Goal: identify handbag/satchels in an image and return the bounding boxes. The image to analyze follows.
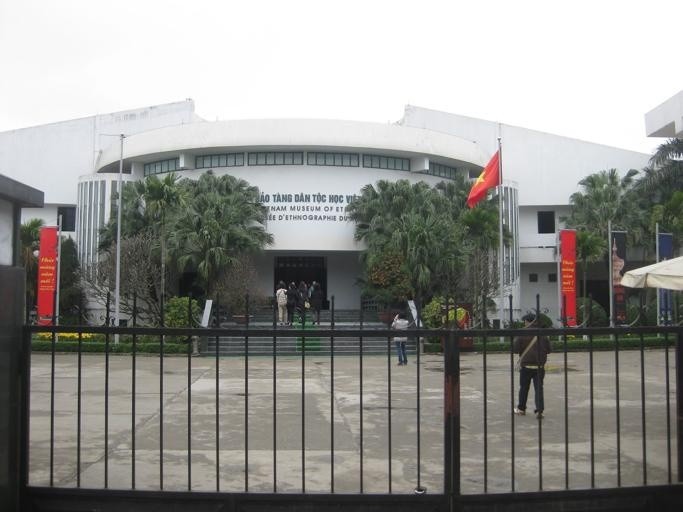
[305,301,311,309]
[514,359,522,372]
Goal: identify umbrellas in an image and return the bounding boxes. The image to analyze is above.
[620,254,682,293]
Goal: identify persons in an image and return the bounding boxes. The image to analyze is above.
[511,314,551,419]
[391,311,408,366]
[276,280,322,328]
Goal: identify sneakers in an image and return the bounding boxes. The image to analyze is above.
[398,361,407,366]
[514,407,545,419]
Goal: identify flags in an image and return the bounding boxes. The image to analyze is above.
[465,148,502,209]
